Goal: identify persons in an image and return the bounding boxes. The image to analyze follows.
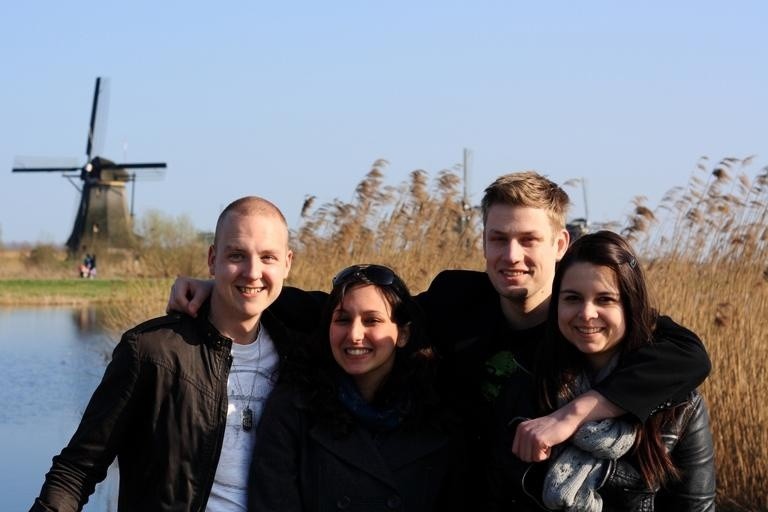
[163,167,714,512]
[78,252,98,279]
[486,226,718,512]
[248,263,494,509]
[26,195,321,512]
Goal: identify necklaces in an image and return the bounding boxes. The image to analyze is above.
[218,324,262,434]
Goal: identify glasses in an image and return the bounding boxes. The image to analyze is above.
[332,263,394,286]
